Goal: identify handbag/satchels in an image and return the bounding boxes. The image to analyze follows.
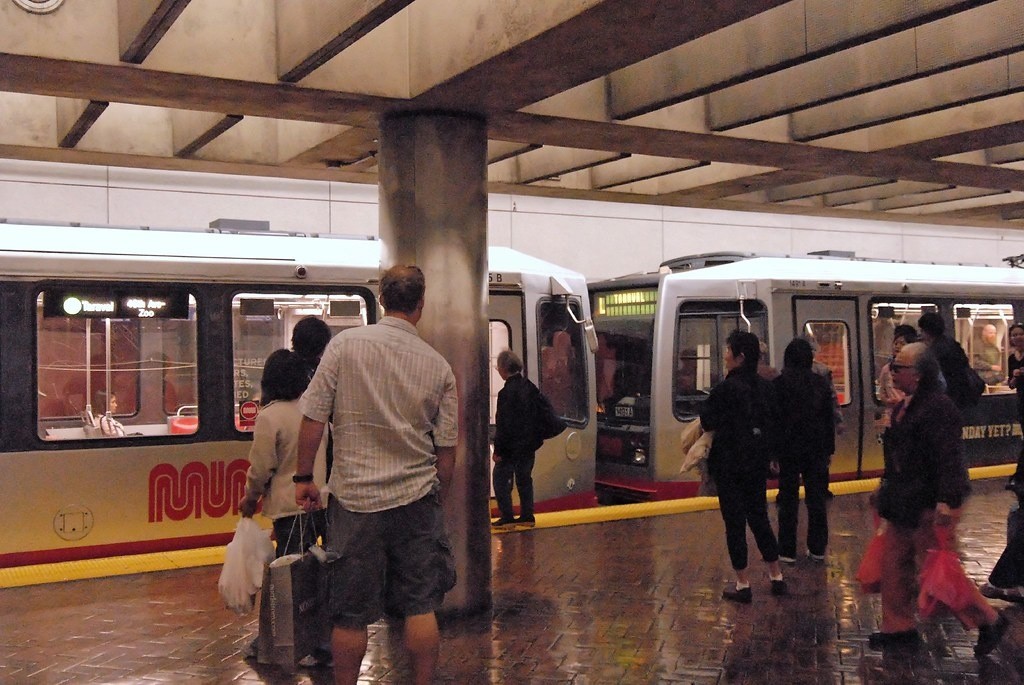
[257,506,328,666]
[917,523,999,630]
[217,516,277,614]
[856,511,890,593]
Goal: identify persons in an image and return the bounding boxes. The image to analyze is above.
[235,349,330,664]
[94,389,142,435]
[774,338,836,563]
[869,342,1009,658]
[292,317,334,483]
[699,331,786,602]
[981,504,1024,602]
[679,350,708,394]
[868,324,917,507]
[1005,325,1024,488]
[969,324,1003,384]
[295,266,458,685]
[492,350,545,528]
[920,312,973,496]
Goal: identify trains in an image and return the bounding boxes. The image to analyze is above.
[0,224,1024,569]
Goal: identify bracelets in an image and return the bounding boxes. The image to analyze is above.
[293,475,313,482]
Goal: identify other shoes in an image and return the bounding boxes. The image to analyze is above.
[806,549,824,564]
[297,654,321,666]
[722,582,753,604]
[981,580,1024,603]
[770,576,787,594]
[974,612,1009,655]
[868,629,918,647]
[516,516,535,526]
[1005,477,1022,489]
[490,517,516,528]
[243,637,258,655]
[777,553,797,565]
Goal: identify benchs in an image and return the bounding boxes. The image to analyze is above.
[78,400,259,444]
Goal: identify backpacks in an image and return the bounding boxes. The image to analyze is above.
[518,379,553,440]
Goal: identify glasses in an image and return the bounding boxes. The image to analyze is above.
[889,364,917,372]
[1008,322,1024,330]
